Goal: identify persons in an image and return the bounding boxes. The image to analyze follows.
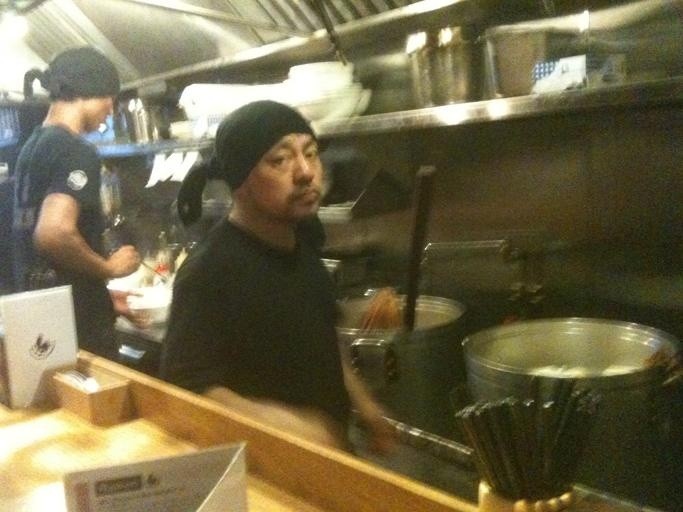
[13,45,143,360]
[158,101,390,452]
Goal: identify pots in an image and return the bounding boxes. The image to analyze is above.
[457,315,683,503]
[337,293,467,420]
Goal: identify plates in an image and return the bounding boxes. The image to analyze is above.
[317,206,354,225]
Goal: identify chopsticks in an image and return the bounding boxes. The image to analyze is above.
[455,374,597,501]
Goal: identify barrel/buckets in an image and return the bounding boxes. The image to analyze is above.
[404,37,492,108]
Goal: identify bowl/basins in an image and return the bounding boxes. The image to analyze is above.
[407,26,584,108]
[129,299,171,326]
[298,80,374,122]
